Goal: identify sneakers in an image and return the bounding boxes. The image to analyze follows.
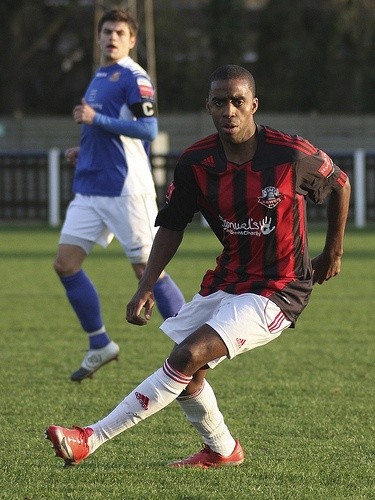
[70,341,120,382]
[166,438,245,469]
[45,423,93,466]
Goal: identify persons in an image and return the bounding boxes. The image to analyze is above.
[45,64,351,469]
[54,9,187,383]
